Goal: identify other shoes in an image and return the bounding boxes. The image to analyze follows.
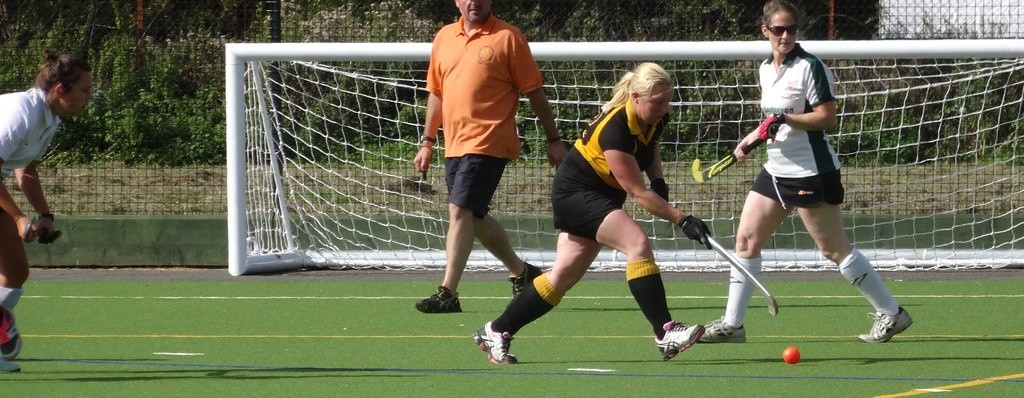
[0,360,21,371]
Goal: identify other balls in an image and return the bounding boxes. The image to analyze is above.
[782,346,801,364]
[705,231,780,318]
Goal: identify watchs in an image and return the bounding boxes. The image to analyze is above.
[420,135,436,143]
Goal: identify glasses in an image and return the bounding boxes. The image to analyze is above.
[766,24,799,36]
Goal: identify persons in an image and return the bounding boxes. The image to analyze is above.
[698,0,913,343]
[0,50,92,373]
[414,0,568,314]
[472,61,706,365]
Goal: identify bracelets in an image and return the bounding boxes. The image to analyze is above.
[421,146,433,152]
[549,137,561,142]
[15,215,26,222]
[38,213,54,221]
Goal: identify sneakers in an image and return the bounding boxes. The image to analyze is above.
[698,315,747,342]
[505,262,543,308]
[415,285,462,314]
[858,305,913,342]
[0,307,22,360]
[654,320,705,362]
[472,321,517,364]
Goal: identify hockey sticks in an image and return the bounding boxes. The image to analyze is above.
[691,124,780,184]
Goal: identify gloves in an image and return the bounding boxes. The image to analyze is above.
[651,178,668,202]
[677,215,713,251]
[758,113,784,141]
